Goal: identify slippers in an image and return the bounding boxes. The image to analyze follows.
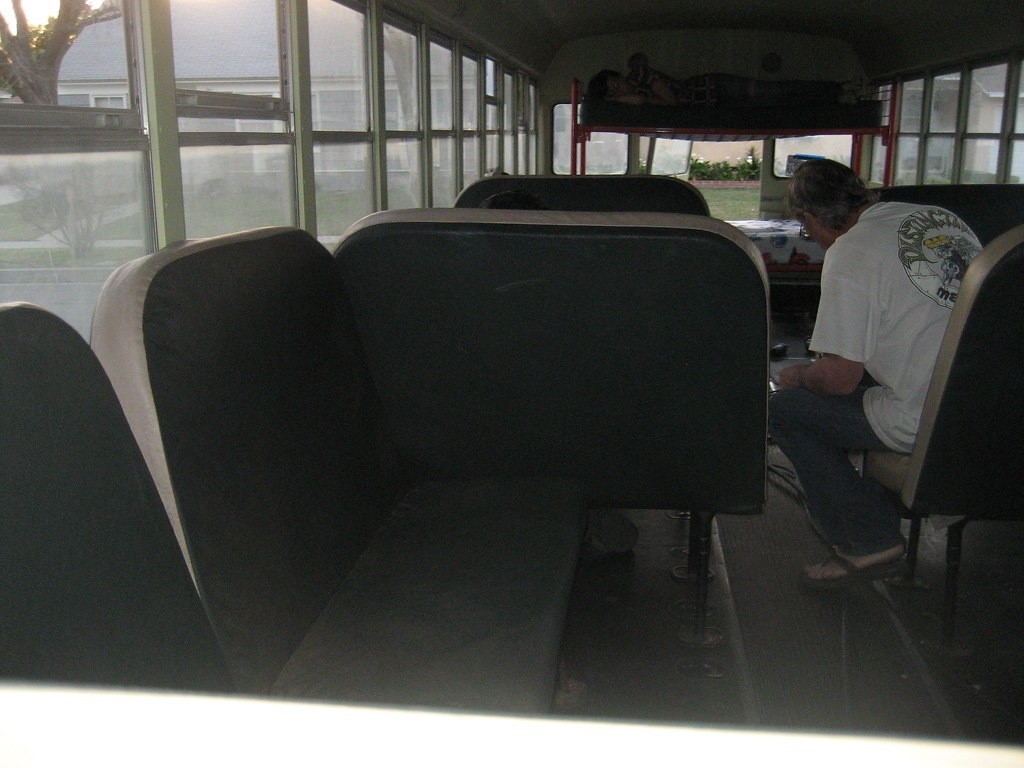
[806,550,909,589]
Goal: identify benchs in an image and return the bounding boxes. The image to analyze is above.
[0,176,773,717]
[862,184,1024,650]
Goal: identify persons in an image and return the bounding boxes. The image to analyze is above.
[766,159,986,567]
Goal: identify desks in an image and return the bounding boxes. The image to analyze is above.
[724,220,828,325]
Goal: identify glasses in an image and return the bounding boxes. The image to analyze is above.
[798,217,813,241]
